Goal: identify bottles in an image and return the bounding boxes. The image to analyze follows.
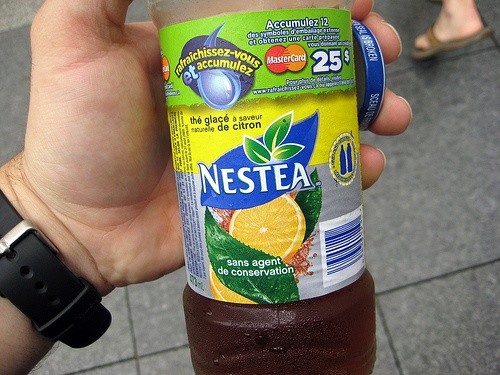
[146,0,378,375]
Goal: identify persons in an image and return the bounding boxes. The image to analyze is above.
[0,0,411,375]
[409,0,492,62]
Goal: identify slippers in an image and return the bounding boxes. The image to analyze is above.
[407,22,493,59]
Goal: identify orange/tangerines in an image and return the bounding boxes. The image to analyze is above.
[210,193,308,305]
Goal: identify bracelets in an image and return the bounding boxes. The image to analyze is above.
[0,190,114,347]
